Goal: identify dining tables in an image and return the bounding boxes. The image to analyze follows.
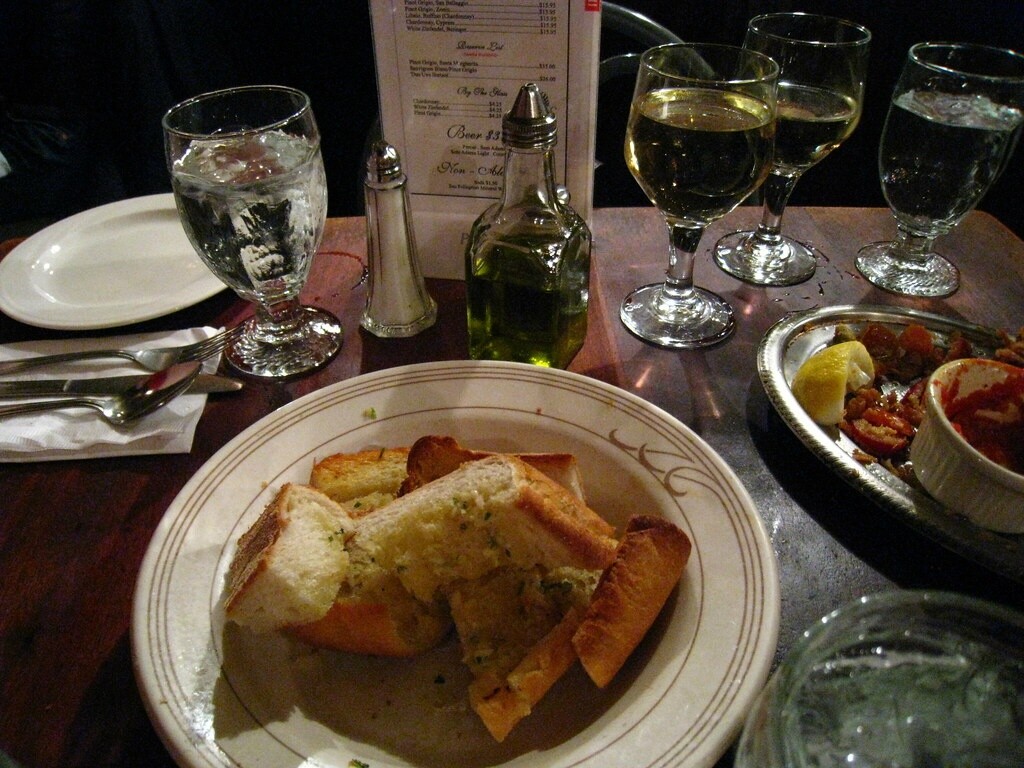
[0,205,1024,768]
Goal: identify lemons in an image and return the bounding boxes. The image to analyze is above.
[792,341,875,424]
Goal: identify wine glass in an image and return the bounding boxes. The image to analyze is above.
[855,44,1024,297]
[711,13,873,289]
[161,85,344,381]
[618,44,780,349]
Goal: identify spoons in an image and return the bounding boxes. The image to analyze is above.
[0,363,204,425]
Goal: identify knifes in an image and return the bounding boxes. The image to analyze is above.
[0,374,243,399]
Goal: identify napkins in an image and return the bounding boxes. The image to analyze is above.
[0,326,227,466]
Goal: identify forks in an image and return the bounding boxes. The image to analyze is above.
[0,319,248,375]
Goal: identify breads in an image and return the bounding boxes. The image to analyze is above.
[222,433,693,744]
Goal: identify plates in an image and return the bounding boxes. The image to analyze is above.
[757,305,1024,582]
[131,360,781,768]
[0,192,229,330]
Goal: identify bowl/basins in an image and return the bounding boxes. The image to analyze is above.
[910,358,1024,534]
[733,586,1024,768]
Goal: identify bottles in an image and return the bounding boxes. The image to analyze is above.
[358,141,438,338]
[463,84,592,370]
